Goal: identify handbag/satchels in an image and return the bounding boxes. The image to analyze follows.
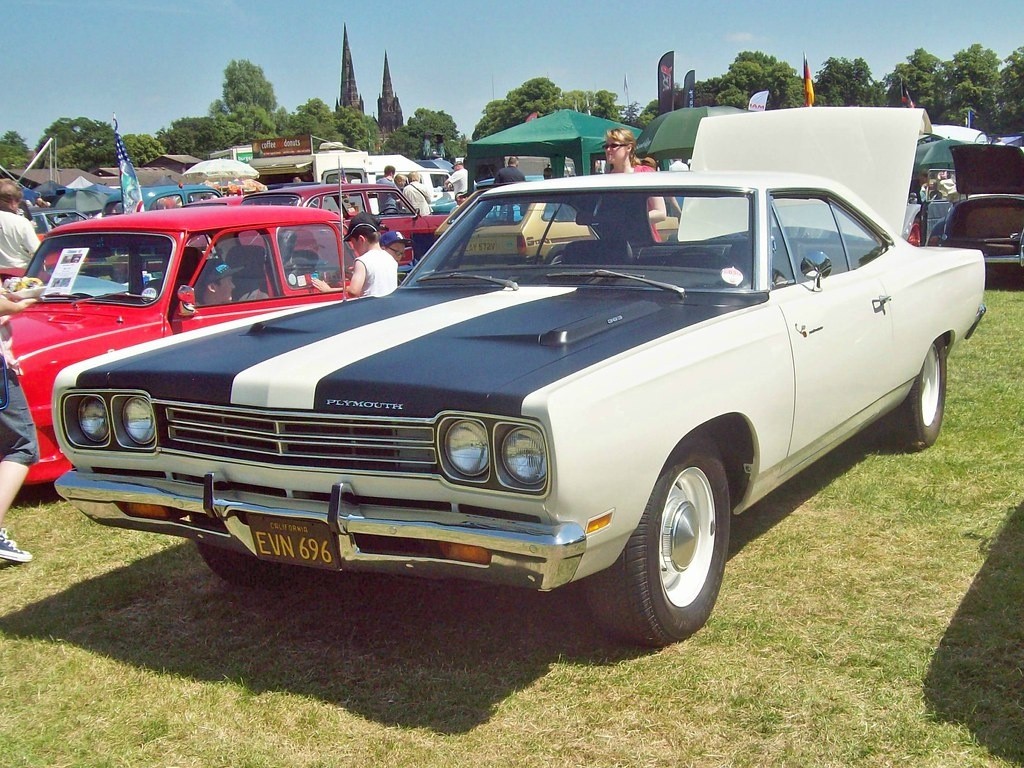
[0,355,10,411]
[424,194,431,205]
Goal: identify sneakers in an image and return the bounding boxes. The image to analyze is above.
[0,528,33,563]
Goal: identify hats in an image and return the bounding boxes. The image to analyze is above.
[380,230,414,250]
[344,211,380,242]
[642,157,657,169]
[196,259,244,287]
[452,161,463,169]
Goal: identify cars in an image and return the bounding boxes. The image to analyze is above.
[2,203,358,489]
[1,172,573,262]
[923,143,1024,266]
[51,106,987,646]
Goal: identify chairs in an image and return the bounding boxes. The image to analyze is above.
[224,244,266,300]
[562,239,635,266]
[160,243,205,312]
[634,242,735,271]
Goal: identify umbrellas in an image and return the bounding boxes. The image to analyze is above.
[182,158,267,197]
[913,138,975,171]
[633,106,752,161]
[30,175,117,213]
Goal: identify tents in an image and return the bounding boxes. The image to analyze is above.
[466,108,644,196]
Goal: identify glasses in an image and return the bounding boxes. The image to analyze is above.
[602,143,629,153]
[384,245,406,258]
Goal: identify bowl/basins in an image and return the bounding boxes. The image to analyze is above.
[3,286,48,301]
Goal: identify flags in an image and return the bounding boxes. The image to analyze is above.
[749,91,769,111]
[804,60,814,107]
[658,51,674,114]
[683,70,695,108]
[114,131,145,214]
[965,109,975,127]
[900,81,914,107]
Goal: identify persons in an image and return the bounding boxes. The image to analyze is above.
[450,192,468,214]
[0,179,43,562]
[293,172,313,182]
[493,156,526,183]
[445,161,476,195]
[576,128,690,243]
[374,165,443,215]
[543,168,552,179]
[165,246,242,304]
[379,231,415,264]
[909,170,951,205]
[343,212,398,297]
